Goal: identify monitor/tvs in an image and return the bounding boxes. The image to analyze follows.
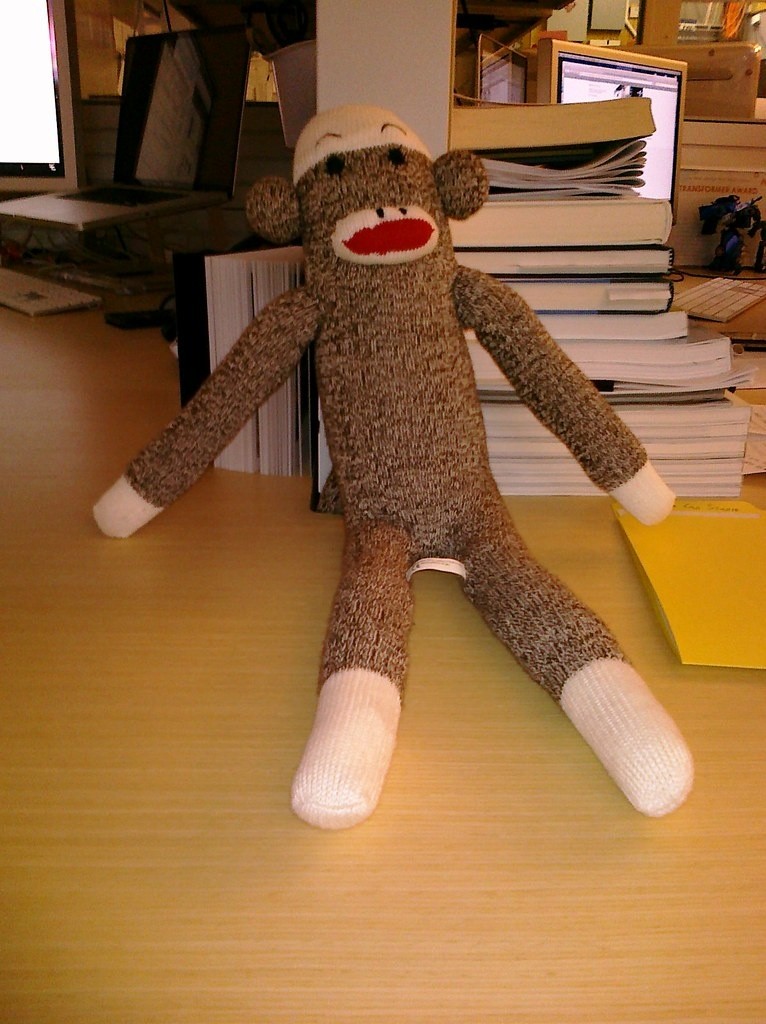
[549,39,688,227]
[473,33,529,108]
[0,0,86,191]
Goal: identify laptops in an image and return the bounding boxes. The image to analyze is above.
[0,23,258,233]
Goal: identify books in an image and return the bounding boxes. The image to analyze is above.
[449,97,758,498]
[171,246,313,479]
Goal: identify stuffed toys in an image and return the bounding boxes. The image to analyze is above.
[93,103,696,831]
